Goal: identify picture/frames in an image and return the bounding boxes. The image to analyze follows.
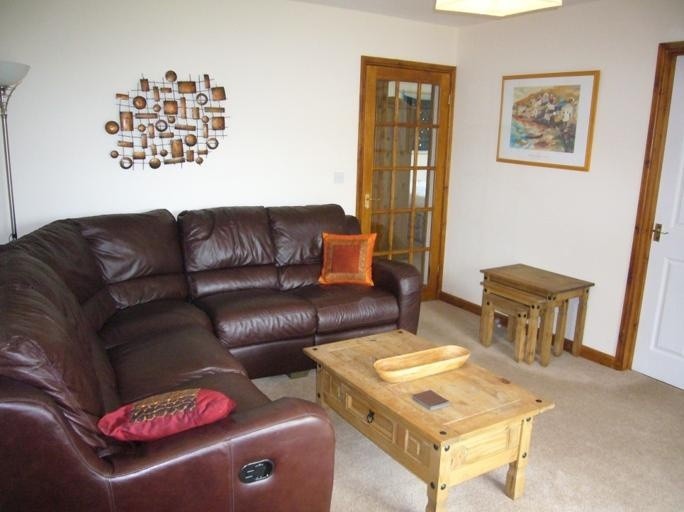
[495,70,599,172]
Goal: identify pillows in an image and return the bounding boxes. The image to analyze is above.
[318,227,376,289]
[97,388,235,440]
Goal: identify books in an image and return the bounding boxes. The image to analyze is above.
[412,389,451,413]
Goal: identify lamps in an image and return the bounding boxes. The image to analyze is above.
[0,61,30,241]
[430,1,563,19]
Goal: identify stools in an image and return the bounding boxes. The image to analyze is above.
[478,264,595,368]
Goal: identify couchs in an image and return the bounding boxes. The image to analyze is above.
[0,210,334,512]
[175,204,425,381]
